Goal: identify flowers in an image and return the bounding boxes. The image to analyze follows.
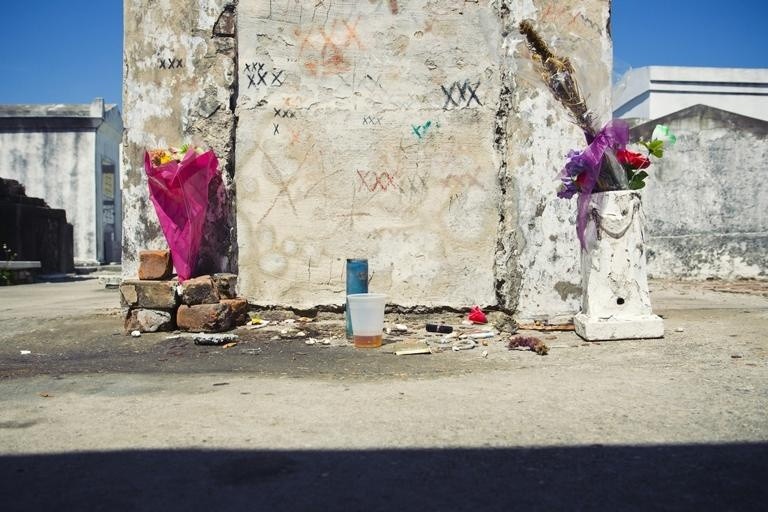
[144,144,219,282]
[557,119,676,199]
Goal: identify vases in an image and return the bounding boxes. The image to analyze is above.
[573,190,664,342]
